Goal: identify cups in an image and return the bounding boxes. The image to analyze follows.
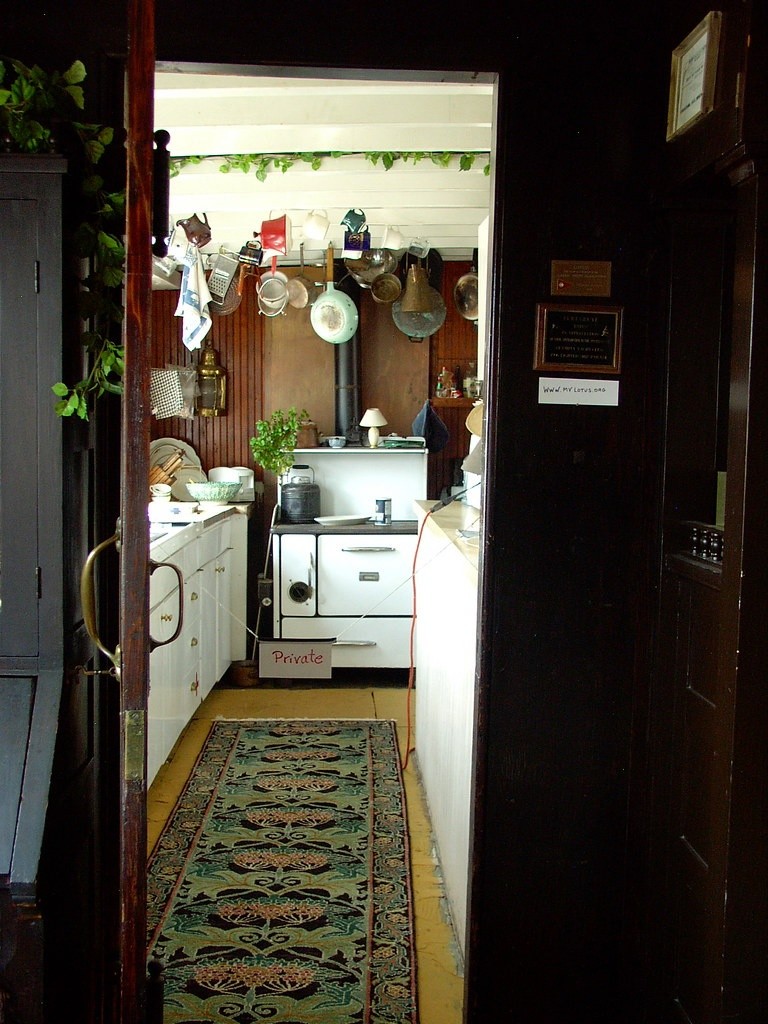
[343,225,372,252]
[153,254,177,277]
[149,483,172,495]
[239,240,264,267]
[176,210,212,248]
[339,208,367,235]
[406,236,432,258]
[164,223,176,248]
[380,225,405,251]
[374,497,393,526]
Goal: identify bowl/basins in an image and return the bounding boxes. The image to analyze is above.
[185,481,244,507]
[151,494,171,502]
[328,437,346,449]
[255,270,289,309]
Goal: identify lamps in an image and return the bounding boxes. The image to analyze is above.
[359,408,388,448]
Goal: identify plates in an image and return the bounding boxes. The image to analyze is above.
[315,515,373,526]
[149,436,207,502]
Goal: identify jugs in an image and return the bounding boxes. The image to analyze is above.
[299,207,330,241]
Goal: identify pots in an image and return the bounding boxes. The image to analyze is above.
[287,242,316,310]
[370,249,403,305]
[310,239,359,345]
[343,247,398,287]
[453,248,478,321]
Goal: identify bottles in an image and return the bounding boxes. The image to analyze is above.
[434,362,484,399]
[278,464,322,524]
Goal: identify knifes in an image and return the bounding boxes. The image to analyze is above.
[161,448,185,479]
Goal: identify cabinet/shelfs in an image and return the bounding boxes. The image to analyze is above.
[272,533,417,668]
[149,517,235,788]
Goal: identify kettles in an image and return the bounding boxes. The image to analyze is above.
[253,210,293,264]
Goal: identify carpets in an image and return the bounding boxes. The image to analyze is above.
[145,717,418,1024]
